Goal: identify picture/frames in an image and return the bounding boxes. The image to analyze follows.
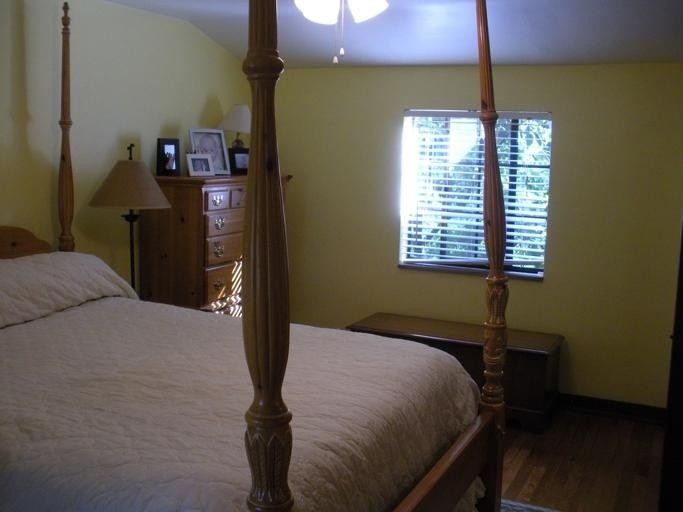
[226,147,251,177]
[154,137,181,179]
[189,129,232,177]
[185,152,216,179]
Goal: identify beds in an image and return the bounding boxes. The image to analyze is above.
[0,1,517,511]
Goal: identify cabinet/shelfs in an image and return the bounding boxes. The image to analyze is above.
[133,174,293,318]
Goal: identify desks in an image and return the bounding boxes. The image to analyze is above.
[344,309,568,424]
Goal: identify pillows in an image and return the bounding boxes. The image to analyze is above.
[0,252,121,329]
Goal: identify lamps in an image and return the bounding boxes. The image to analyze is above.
[215,102,254,146]
[290,0,391,72]
[87,143,175,299]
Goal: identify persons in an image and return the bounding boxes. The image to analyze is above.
[193,159,210,171]
[165,150,175,169]
[198,135,221,170]
